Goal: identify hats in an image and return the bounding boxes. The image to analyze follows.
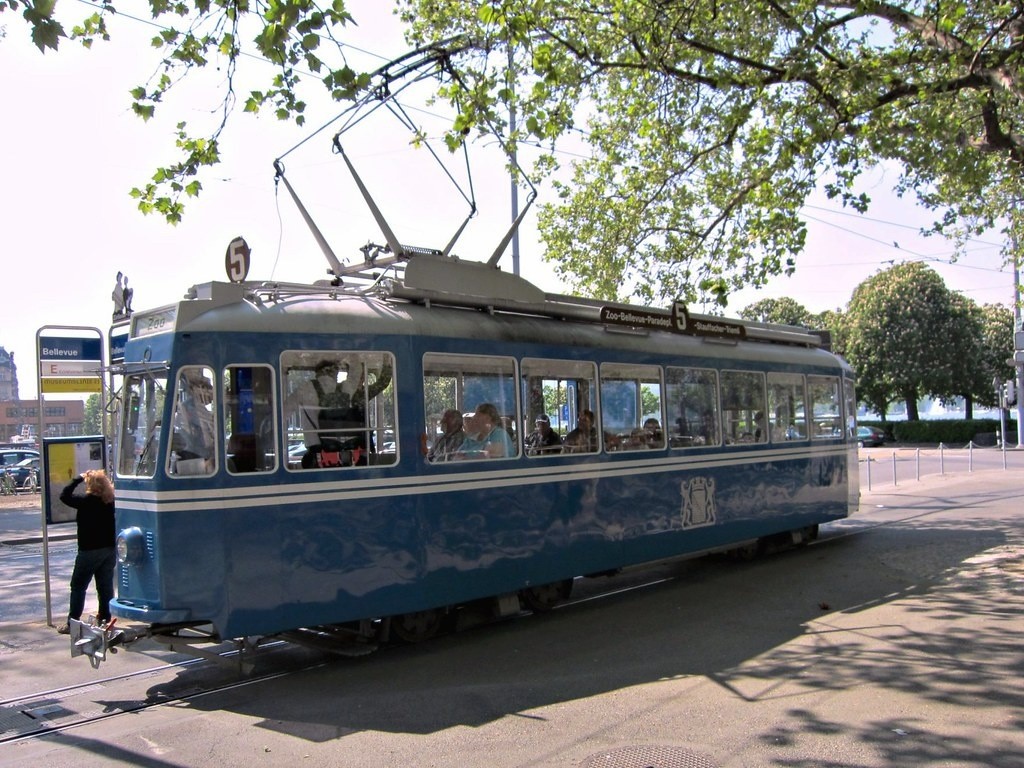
[535,414,549,422]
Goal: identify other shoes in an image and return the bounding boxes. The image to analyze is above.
[57,624,71,634]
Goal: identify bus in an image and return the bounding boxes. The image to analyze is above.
[69,237,861,680]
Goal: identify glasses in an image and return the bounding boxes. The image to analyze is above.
[577,418,589,424]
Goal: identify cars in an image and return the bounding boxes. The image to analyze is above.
[0,457,40,491]
[857,425,886,448]
[0,450,40,469]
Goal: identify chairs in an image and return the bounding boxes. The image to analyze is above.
[227,433,266,472]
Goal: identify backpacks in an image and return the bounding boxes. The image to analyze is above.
[303,380,359,453]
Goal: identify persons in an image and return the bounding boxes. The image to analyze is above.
[787,423,794,440]
[428,402,517,462]
[524,414,563,456]
[669,410,715,447]
[57,469,116,633]
[604,418,665,451]
[174,377,216,475]
[564,409,598,453]
[285,351,393,468]
[725,411,766,444]
